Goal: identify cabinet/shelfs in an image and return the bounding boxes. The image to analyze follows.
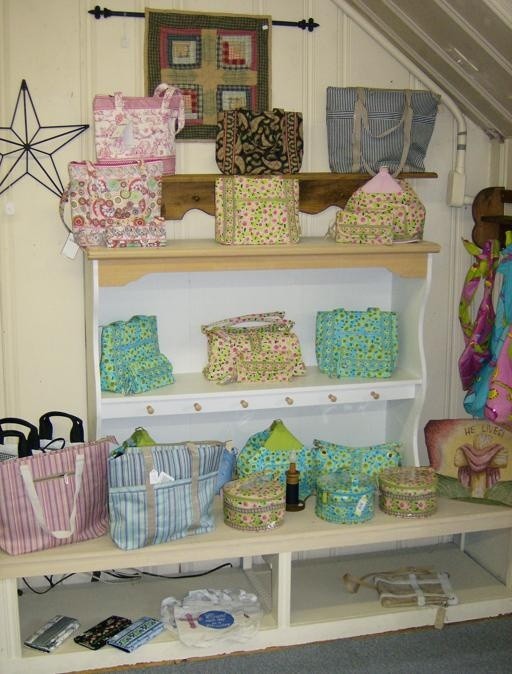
[82,238,441,469]
[0,491,512,674]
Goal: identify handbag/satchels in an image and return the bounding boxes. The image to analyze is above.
[213,103,305,246]
[315,466,378,523]
[378,467,442,517]
[343,565,459,629]
[214,438,236,496]
[462,250,511,428]
[236,419,316,504]
[107,426,223,550]
[219,469,287,533]
[200,310,308,384]
[66,94,185,250]
[312,436,404,506]
[326,85,442,246]
[99,314,173,394]
[1,411,119,555]
[316,306,400,379]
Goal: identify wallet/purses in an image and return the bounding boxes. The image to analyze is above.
[73,615,166,654]
[24,614,81,653]
